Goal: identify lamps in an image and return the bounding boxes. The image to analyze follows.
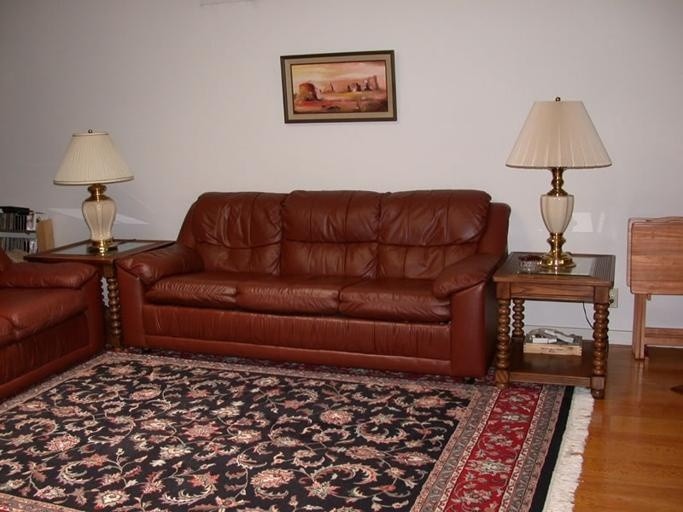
[504,95,613,270]
[54,130,135,251]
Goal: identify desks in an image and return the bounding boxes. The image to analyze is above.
[20,239,175,353]
[489,249,617,399]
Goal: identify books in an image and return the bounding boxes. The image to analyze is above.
[0,205,45,260]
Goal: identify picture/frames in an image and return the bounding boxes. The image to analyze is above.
[278,54,398,125]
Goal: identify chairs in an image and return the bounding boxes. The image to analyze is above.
[0,249,106,406]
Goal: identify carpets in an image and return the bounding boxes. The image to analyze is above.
[0,346,595,510]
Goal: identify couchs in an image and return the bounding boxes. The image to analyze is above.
[114,190,510,384]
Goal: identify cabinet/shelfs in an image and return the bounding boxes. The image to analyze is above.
[0,220,54,255]
